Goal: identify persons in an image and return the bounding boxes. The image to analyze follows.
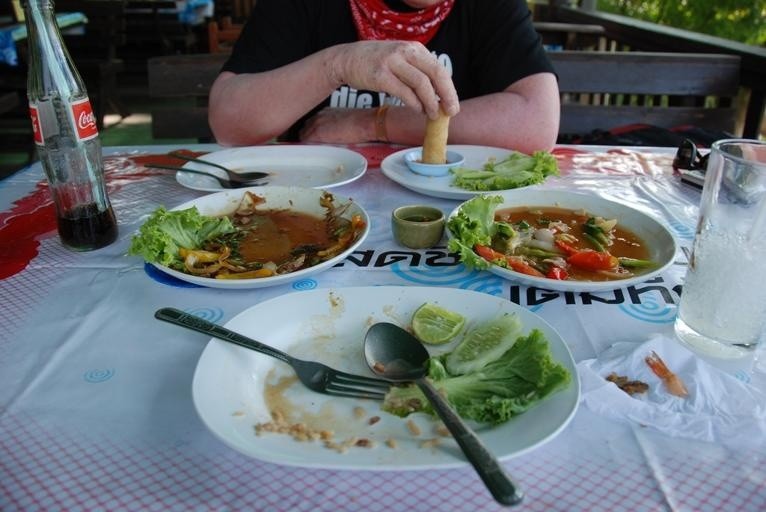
[205,1,564,158]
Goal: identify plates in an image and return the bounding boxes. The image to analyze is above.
[175,145,369,192]
[380,144,550,202]
[146,187,370,291]
[192,285,583,474]
[445,185,679,293]
[403,148,462,175]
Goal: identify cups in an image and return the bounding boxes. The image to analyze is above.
[391,207,444,247]
[673,136,766,356]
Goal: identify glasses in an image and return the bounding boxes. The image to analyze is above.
[673,138,743,175]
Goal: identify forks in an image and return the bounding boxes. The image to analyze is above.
[153,305,393,401]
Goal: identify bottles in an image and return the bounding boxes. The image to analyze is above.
[17,0,120,254]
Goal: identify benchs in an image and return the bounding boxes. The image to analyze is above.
[146,53,742,145]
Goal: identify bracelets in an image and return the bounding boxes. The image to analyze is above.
[375,101,393,145]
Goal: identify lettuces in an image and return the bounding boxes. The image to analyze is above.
[448,150,561,190]
[128,203,234,271]
[445,193,505,272]
[380,329,571,424]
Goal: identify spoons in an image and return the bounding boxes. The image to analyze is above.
[363,320,525,506]
[142,152,271,188]
